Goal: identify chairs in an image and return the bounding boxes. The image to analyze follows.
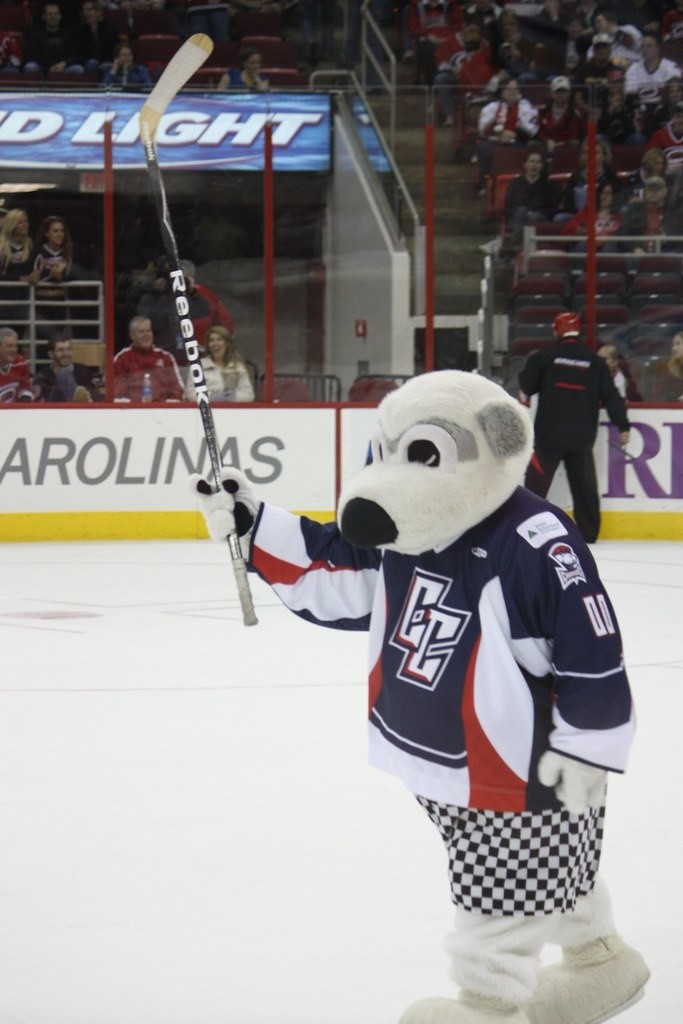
[0,0,683,403]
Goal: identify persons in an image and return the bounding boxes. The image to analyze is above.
[521,308,632,547]
[190,369,652,1023]
[0,0,683,406]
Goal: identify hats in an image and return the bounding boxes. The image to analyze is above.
[550,75,570,92]
[592,33,609,46]
[645,176,666,189]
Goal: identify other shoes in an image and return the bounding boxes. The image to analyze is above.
[479,237,504,257]
[445,115,454,126]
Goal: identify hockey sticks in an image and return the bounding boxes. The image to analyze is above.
[131,30,262,632]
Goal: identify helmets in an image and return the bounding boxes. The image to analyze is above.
[551,313,583,338]
